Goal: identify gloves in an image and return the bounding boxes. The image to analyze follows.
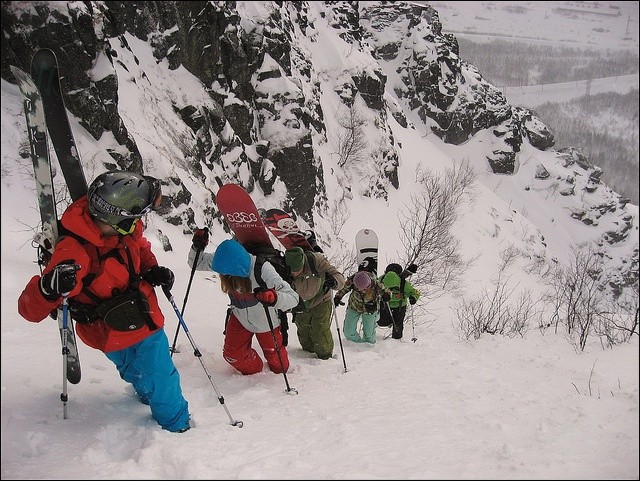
[255,288,277,306]
[145,264,175,290]
[409,295,417,305]
[382,292,391,301]
[333,295,345,308]
[192,229,209,249]
[38,258,82,302]
[325,272,339,289]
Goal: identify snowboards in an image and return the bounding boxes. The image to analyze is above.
[354,227,379,274]
[262,206,315,251]
[216,183,274,248]
[401,262,418,279]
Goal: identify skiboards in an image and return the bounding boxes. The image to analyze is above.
[9,47,88,384]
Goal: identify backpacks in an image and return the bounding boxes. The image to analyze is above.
[284,246,346,361]
[244,247,306,315]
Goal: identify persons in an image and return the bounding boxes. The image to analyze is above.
[187,226,300,376]
[377,272,420,340]
[16,169,192,434]
[334,270,393,344]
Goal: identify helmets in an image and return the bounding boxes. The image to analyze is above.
[86,170,162,235]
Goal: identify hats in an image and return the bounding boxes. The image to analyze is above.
[352,271,371,290]
[285,246,305,272]
